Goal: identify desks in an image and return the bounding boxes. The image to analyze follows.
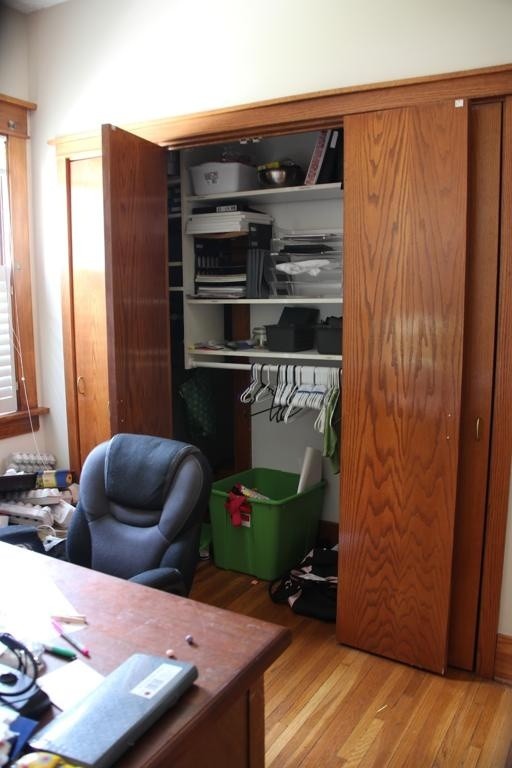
[0,541,292,768]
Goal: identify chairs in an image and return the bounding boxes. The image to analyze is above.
[0,432,214,597]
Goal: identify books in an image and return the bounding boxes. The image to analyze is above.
[181,208,274,235]
[27,652,199,768]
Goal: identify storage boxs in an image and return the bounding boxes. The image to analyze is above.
[206,462,327,584]
[314,313,343,357]
[264,304,320,356]
[189,160,259,196]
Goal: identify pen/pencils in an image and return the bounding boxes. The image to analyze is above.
[41,615,88,658]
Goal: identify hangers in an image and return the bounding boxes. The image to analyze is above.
[239,358,340,435]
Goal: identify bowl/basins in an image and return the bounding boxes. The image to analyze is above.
[257,167,300,188]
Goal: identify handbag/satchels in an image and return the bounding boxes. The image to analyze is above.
[174,368,234,441]
[269,545,338,621]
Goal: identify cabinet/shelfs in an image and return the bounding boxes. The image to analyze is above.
[46,61,512,680]
